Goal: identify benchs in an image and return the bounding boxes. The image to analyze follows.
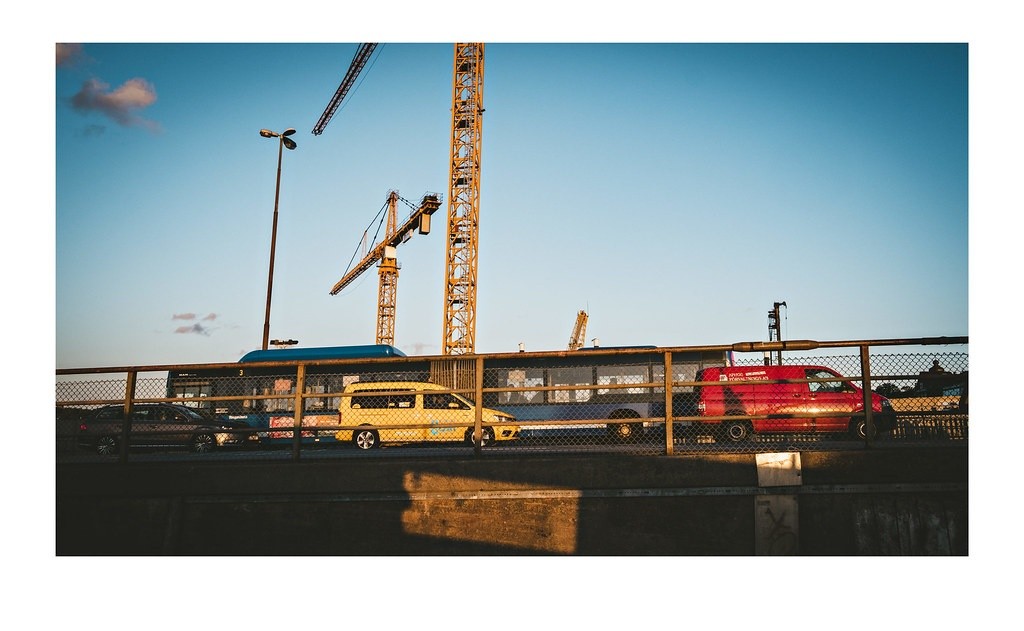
[499,392,542,404]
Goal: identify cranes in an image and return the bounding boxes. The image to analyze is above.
[329,188,444,346]
[567,309,588,351]
[311,43,488,356]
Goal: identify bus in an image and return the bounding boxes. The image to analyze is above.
[168,346,731,449]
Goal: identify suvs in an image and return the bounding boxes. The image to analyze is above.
[76,402,253,456]
[334,379,522,450]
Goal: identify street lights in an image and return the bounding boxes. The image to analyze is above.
[259,127,298,350]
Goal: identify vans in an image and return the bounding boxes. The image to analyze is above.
[694,365,898,446]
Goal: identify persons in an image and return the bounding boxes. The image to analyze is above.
[434,396,445,408]
[505,378,623,403]
